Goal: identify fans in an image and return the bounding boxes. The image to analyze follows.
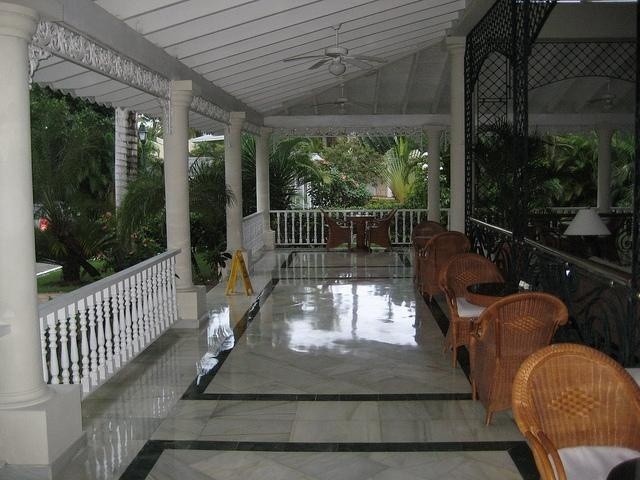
[282,22,386,112]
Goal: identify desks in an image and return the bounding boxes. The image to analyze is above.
[466,280,552,310]
[344,215,372,253]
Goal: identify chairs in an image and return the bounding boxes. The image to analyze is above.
[419,231,473,301]
[411,220,446,280]
[320,209,351,253]
[366,209,398,252]
[469,293,570,428]
[511,343,639,480]
[440,252,505,369]
